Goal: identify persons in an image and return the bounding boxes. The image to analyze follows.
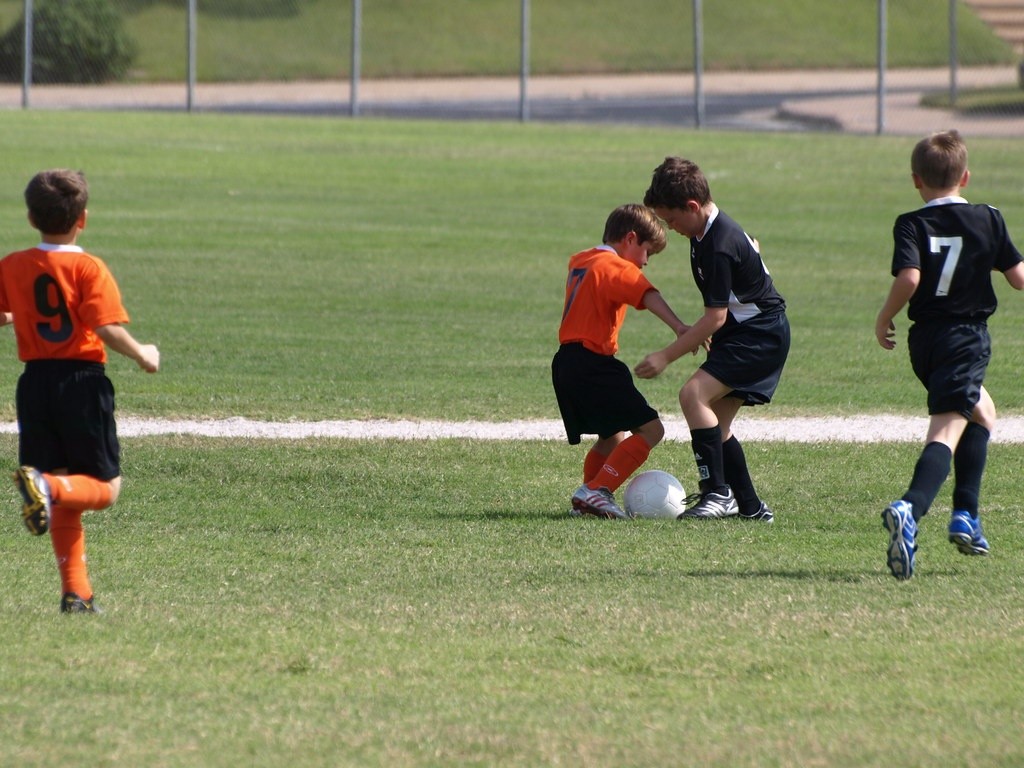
[875,130,1024,582]
[1,170,160,615]
[634,155,790,523]
[552,203,711,519]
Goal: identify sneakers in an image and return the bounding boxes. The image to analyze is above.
[881,500,920,581]
[678,484,739,520]
[60,592,99,612]
[572,484,629,520]
[948,510,991,556]
[731,502,773,523]
[15,466,51,536]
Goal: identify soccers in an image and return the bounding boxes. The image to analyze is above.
[623,470,687,520]
